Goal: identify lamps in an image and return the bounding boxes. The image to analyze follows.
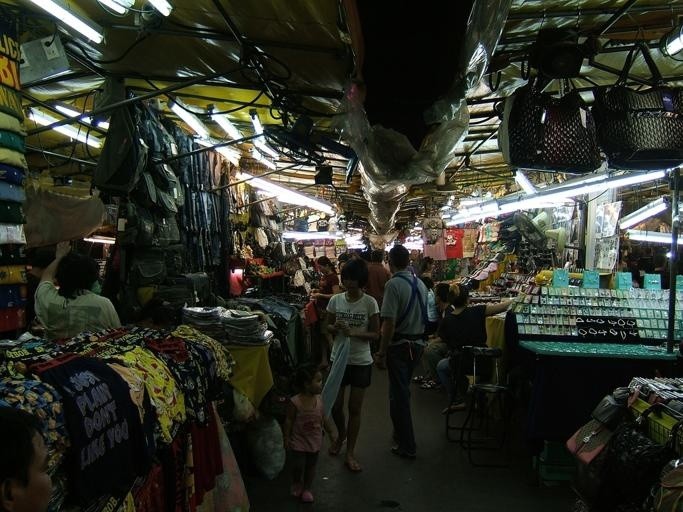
[83,233,117,245]
[95,0,129,17]
[659,22,683,57]
[248,147,277,171]
[249,138,281,162]
[193,134,242,169]
[234,170,337,218]
[623,229,683,245]
[618,194,671,230]
[385,234,425,254]
[206,103,245,145]
[23,104,103,151]
[278,231,344,240]
[344,227,368,252]
[147,0,174,17]
[46,98,110,131]
[509,167,537,196]
[440,191,499,228]
[30,0,108,50]
[498,163,683,213]
[167,94,210,141]
[248,108,267,145]
[413,221,424,231]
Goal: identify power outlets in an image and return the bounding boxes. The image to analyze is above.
[16,32,72,87]
[148,97,165,112]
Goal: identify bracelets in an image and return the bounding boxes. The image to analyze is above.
[629,330,638,336]
[596,318,605,324]
[609,328,618,336]
[586,318,595,322]
[588,327,598,336]
[608,320,618,327]
[579,328,587,336]
[618,319,626,328]
[598,329,608,336]
[620,330,627,340]
[627,320,635,328]
[576,317,584,322]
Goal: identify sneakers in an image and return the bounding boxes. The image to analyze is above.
[441,401,468,415]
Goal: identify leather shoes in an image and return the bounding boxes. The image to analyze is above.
[396,448,417,460]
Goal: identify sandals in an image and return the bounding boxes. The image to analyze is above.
[302,492,313,502]
[291,484,303,496]
[412,376,424,383]
[420,380,437,389]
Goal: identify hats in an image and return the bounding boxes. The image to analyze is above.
[257,390,299,419]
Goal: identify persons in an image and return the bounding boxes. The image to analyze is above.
[33,240,122,341]
[372,239,430,460]
[1,406,53,512]
[440,283,519,414]
[338,254,349,288]
[308,257,342,373]
[383,251,390,271]
[323,256,382,474]
[363,251,393,311]
[412,284,459,390]
[419,275,438,340]
[279,366,340,505]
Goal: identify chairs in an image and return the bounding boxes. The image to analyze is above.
[467,344,508,466]
[445,346,495,445]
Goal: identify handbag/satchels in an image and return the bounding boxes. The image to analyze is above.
[142,119,165,163]
[155,186,178,218]
[593,396,627,430]
[653,463,683,512]
[566,419,616,471]
[170,178,185,207]
[285,259,300,275]
[302,270,314,282]
[304,301,318,327]
[291,242,305,259]
[143,100,179,165]
[152,212,180,247]
[297,254,314,269]
[303,282,312,294]
[151,159,178,193]
[269,199,284,223]
[306,266,322,281]
[90,100,149,198]
[294,269,305,287]
[497,71,602,174]
[252,193,264,215]
[254,210,270,228]
[265,226,285,243]
[609,402,683,484]
[138,171,156,208]
[127,251,176,287]
[271,241,294,263]
[174,272,212,307]
[146,289,196,325]
[167,244,188,278]
[642,459,682,512]
[591,39,683,170]
[259,196,273,215]
[124,201,155,248]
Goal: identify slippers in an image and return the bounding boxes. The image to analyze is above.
[344,458,363,473]
[328,442,343,456]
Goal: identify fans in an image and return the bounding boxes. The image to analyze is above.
[263,113,359,185]
[512,211,568,252]
